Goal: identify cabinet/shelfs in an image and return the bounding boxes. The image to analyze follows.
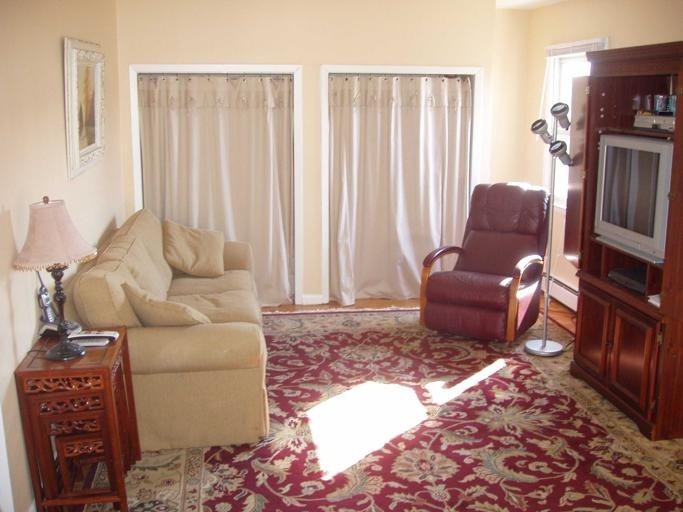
[13,325,144,512]
[570,41,682,439]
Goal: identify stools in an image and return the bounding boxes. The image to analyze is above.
[51,429,115,497]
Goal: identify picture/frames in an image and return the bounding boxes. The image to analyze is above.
[62,38,107,181]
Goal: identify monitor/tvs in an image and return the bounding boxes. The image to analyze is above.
[593,134,674,264]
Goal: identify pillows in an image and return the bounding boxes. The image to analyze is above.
[162,216,225,279]
[119,280,212,329]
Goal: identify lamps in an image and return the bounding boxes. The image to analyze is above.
[523,101,574,358]
[11,196,100,361]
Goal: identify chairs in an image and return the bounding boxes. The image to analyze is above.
[415,179,551,344]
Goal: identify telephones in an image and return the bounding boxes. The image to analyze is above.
[35,284,82,337]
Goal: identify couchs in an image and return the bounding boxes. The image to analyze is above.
[53,207,270,454]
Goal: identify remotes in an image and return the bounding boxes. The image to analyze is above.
[67,331,120,340]
[70,338,109,346]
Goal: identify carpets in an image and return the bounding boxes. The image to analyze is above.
[42,304,683,511]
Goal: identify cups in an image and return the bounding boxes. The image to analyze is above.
[631,92,677,113]
